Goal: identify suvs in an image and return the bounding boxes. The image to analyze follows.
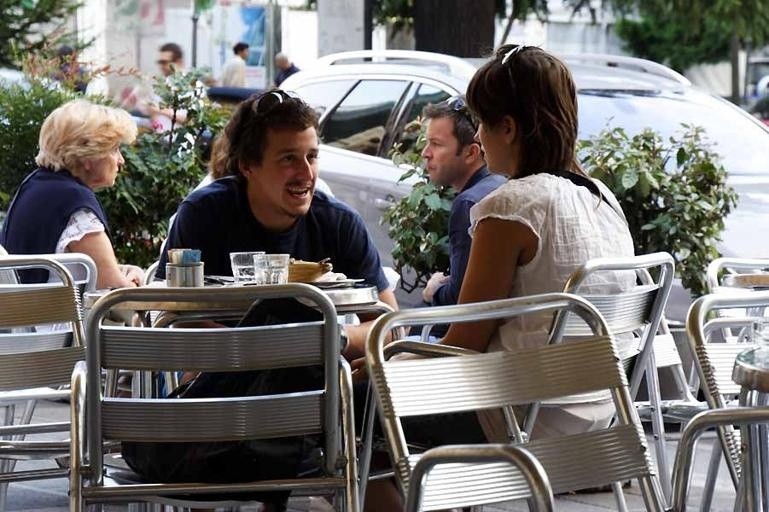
[273,48,768,342]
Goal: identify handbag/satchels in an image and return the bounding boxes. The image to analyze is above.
[121,297,323,503]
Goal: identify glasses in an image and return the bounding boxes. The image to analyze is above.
[503,45,545,93]
[245,91,300,121]
[446,94,479,132]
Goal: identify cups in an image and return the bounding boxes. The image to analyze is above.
[230,252,265,284]
[164,261,205,289]
[253,254,289,285]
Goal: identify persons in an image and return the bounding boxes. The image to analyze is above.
[53,43,300,128]
[156,88,403,512]
[1,98,145,336]
[363,43,639,511]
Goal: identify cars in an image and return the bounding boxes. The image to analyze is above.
[0,70,195,156]
[750,96,769,127]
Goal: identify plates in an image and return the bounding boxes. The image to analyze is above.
[302,279,365,289]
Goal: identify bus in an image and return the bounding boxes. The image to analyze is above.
[748,56,769,98]
